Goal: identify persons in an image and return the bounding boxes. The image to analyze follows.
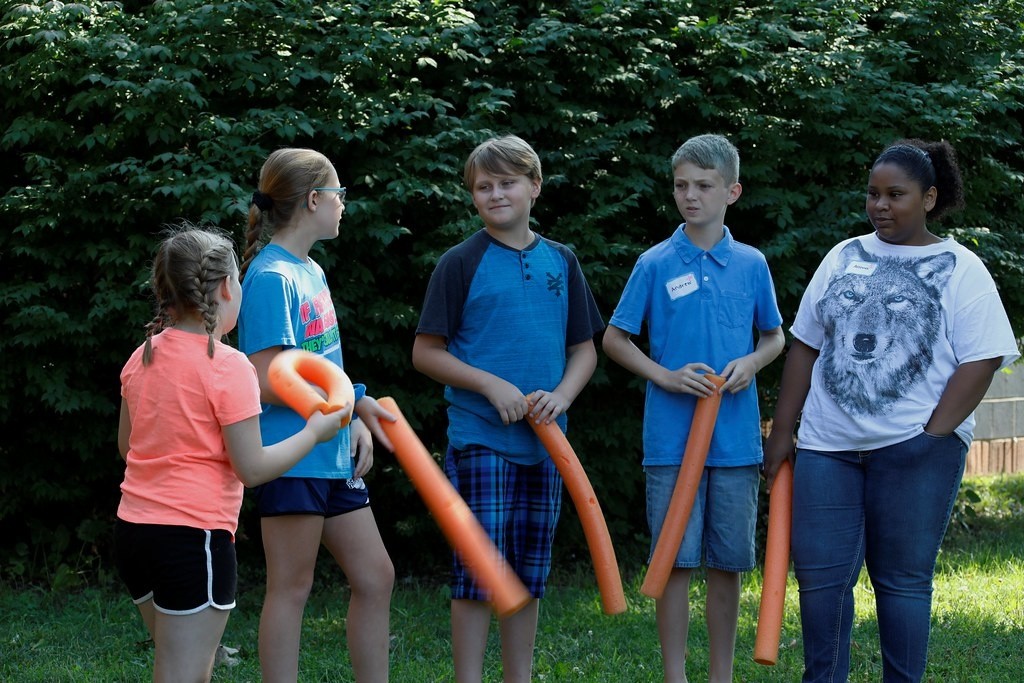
[762,138,1022,683]
[115,229,351,683]
[238,147,397,683]
[411,134,608,683]
[602,135,786,683]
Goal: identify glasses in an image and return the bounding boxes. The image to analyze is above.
[302,187,346,209]
